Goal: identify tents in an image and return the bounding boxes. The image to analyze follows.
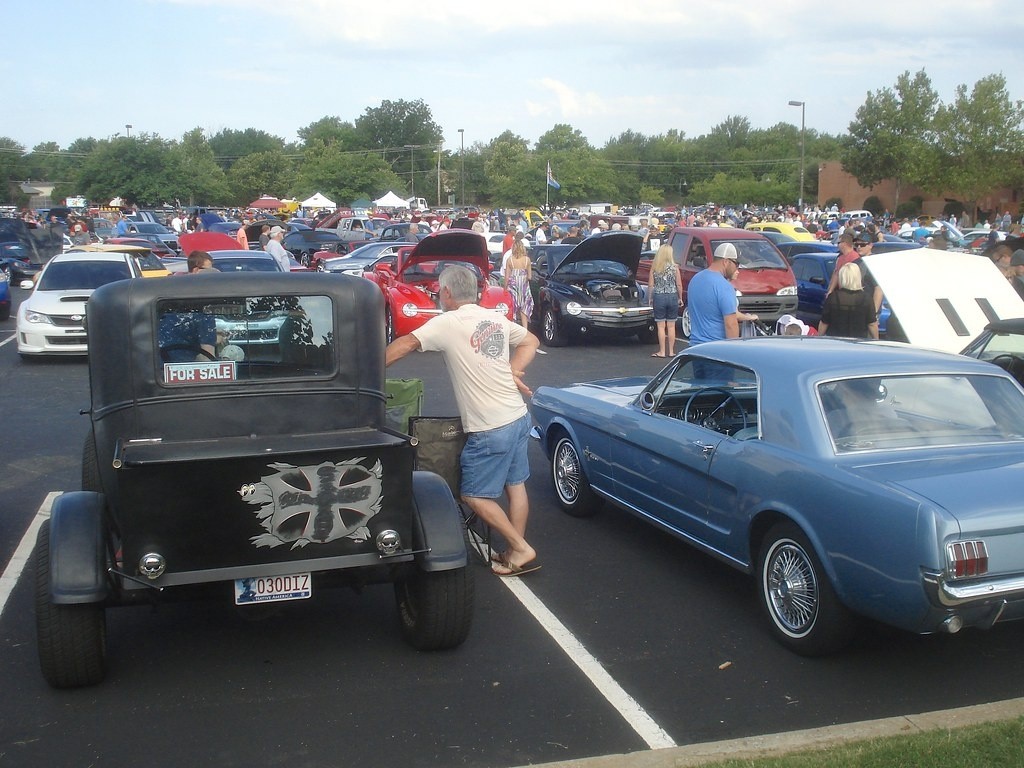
[373,190,410,209]
[302,192,336,209]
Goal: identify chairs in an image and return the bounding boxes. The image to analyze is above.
[840,417,919,438]
[277,316,323,367]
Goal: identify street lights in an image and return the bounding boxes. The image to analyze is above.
[458,129,465,212]
[433,150,440,207]
[404,144,420,201]
[126,125,132,138]
[789,101,806,214]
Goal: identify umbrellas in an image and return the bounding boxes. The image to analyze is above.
[277,199,300,214]
[346,197,378,208]
[250,194,286,211]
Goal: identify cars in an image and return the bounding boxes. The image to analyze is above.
[530,336,1024,656]
[34,270,474,687]
[522,202,814,244]
[0,206,188,321]
[635,210,1009,334]
[16,252,143,362]
[489,230,657,348]
[861,247,1024,392]
[178,206,514,343]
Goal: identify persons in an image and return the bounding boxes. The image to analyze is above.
[0,201,972,252]
[384,266,541,576]
[267,226,290,272]
[649,244,684,357]
[975,211,1024,301]
[827,378,898,439]
[818,227,883,340]
[775,314,818,336]
[161,251,232,364]
[688,243,759,349]
[499,226,534,329]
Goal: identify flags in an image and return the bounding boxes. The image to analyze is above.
[547,167,560,190]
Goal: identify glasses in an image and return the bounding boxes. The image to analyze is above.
[997,256,1003,263]
[838,241,841,245]
[436,286,446,299]
[855,242,869,247]
[729,258,739,267]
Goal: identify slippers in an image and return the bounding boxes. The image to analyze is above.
[651,353,666,358]
[494,561,542,577]
[491,549,506,563]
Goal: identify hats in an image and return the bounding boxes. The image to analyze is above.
[1010,249,1024,266]
[75,225,81,231]
[271,226,286,233]
[508,226,519,230]
[852,233,873,242]
[714,242,743,259]
[242,220,252,225]
